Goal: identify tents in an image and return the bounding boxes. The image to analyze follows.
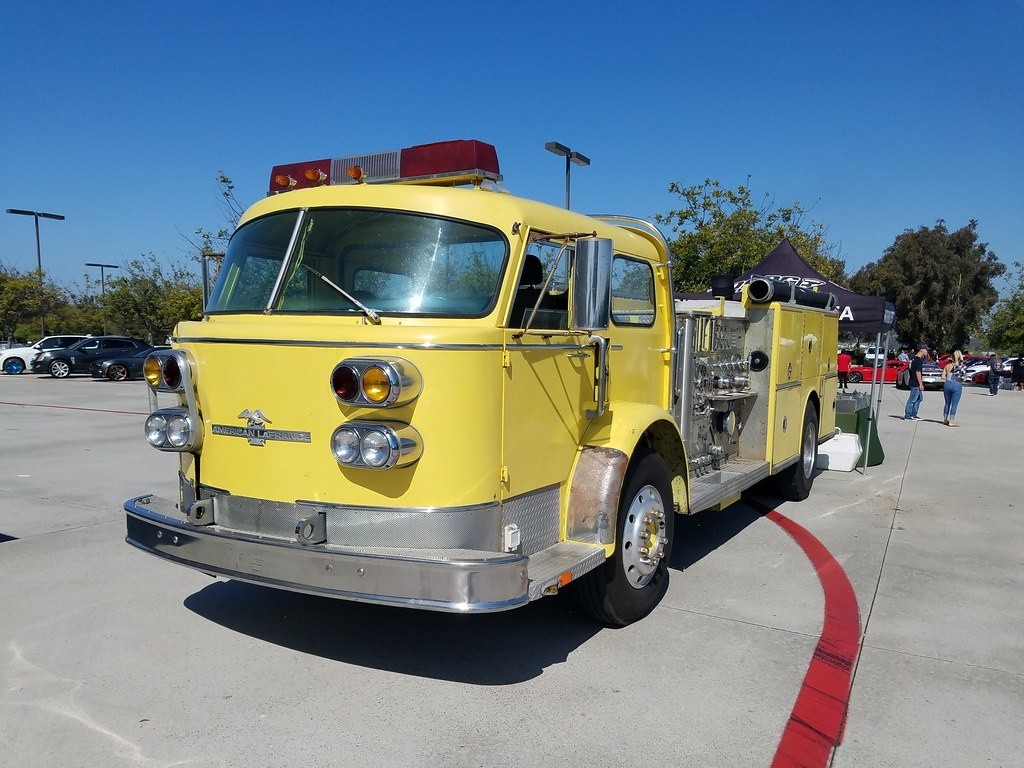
[696,238,894,476]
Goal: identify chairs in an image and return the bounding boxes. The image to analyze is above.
[509,255,543,324]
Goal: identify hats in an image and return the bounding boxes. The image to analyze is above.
[919,344,931,350]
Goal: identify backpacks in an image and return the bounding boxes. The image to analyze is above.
[992,359,1003,371]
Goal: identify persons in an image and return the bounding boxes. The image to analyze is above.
[887,349,938,364]
[1011,353,1024,391]
[837,349,851,389]
[905,345,931,421]
[941,350,966,427]
[987,354,1003,396]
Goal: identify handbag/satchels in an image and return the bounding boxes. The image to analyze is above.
[816,426,863,472]
[953,365,967,379]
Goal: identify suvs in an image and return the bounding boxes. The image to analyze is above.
[0,334,99,375]
[864,347,888,367]
[30,335,151,378]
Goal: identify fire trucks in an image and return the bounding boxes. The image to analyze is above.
[125,138,840,628]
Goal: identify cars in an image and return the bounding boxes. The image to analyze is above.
[965,357,1024,381]
[848,360,908,384]
[89,344,172,381]
[936,354,953,368]
[972,370,1011,385]
[896,363,946,390]
[955,358,988,378]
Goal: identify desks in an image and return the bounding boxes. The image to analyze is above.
[834,406,885,467]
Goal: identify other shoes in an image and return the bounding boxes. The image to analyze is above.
[944,420,959,427]
[838,386,848,389]
[1012,387,1024,391]
[987,394,998,396]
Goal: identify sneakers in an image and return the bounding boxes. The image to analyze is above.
[905,416,923,422]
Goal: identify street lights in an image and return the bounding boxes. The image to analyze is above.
[5,209,65,338]
[545,141,591,211]
[85,263,118,336]
